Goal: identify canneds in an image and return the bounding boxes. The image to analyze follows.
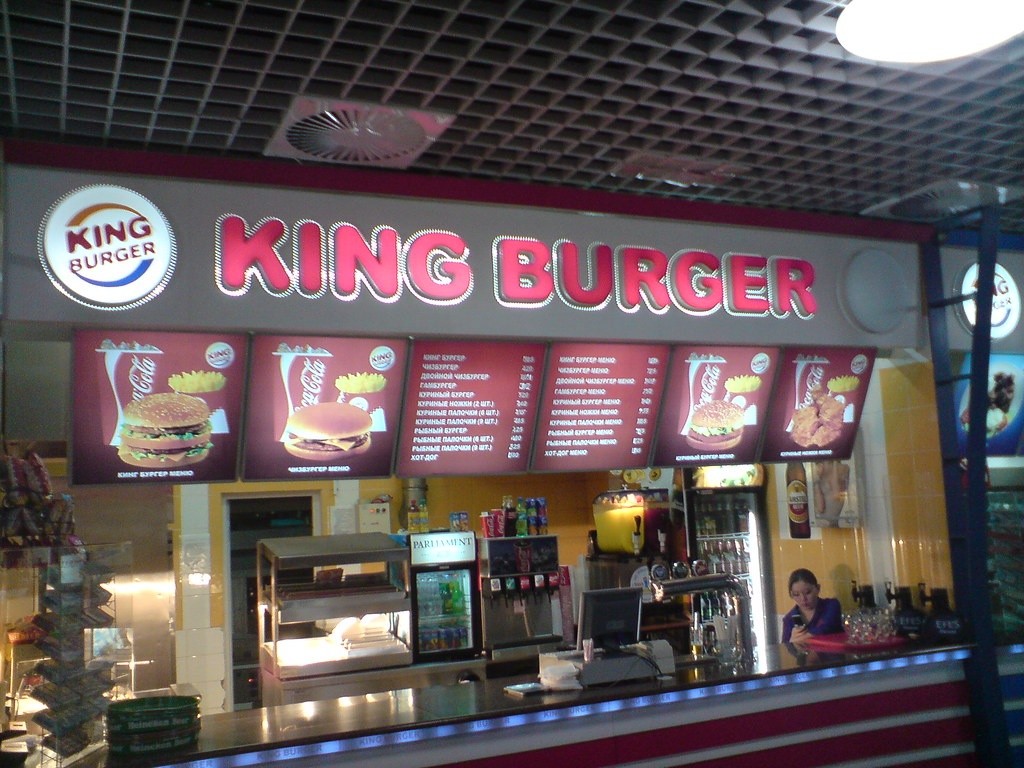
[419,625,468,650]
[448,512,469,532]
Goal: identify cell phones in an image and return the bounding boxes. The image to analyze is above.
[792,615,804,628]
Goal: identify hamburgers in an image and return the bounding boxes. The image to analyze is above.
[116,392,214,467]
[284,402,372,460]
[687,401,744,451]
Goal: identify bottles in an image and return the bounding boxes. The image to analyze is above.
[408,499,421,534]
[689,666,705,684]
[786,463,812,539]
[525,496,538,535]
[419,499,430,534]
[690,590,745,658]
[693,500,751,574]
[536,496,548,534]
[516,497,528,536]
[501,495,516,537]
[417,574,468,652]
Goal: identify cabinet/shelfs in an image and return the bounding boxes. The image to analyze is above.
[0,541,134,768]
[257,532,414,680]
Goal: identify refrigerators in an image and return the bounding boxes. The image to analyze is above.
[607,461,770,662]
[388,528,480,665]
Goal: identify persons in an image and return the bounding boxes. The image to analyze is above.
[782,568,844,642]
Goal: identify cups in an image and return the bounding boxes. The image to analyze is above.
[677,361,733,436]
[272,351,335,443]
[783,360,832,433]
[491,509,506,537]
[479,514,495,537]
[95,349,167,445]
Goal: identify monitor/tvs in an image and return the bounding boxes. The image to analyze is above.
[577,586,643,650]
